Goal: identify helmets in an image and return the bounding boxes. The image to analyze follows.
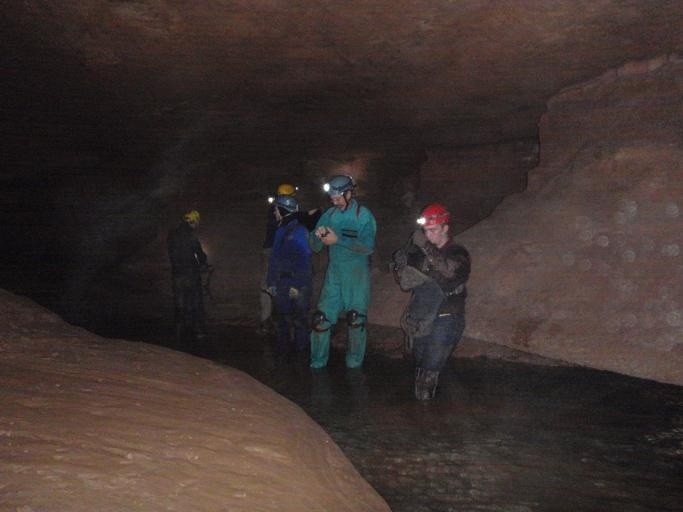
[420,204,451,227]
[183,209,200,228]
[329,175,353,196]
[272,184,298,213]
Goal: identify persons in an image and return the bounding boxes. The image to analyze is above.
[166,207,207,328]
[392,201,471,402]
[308,174,377,369]
[266,195,312,353]
[260,184,325,328]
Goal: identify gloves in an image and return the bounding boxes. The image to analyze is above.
[394,248,407,267]
[412,230,427,248]
[266,286,277,296]
[289,288,298,298]
